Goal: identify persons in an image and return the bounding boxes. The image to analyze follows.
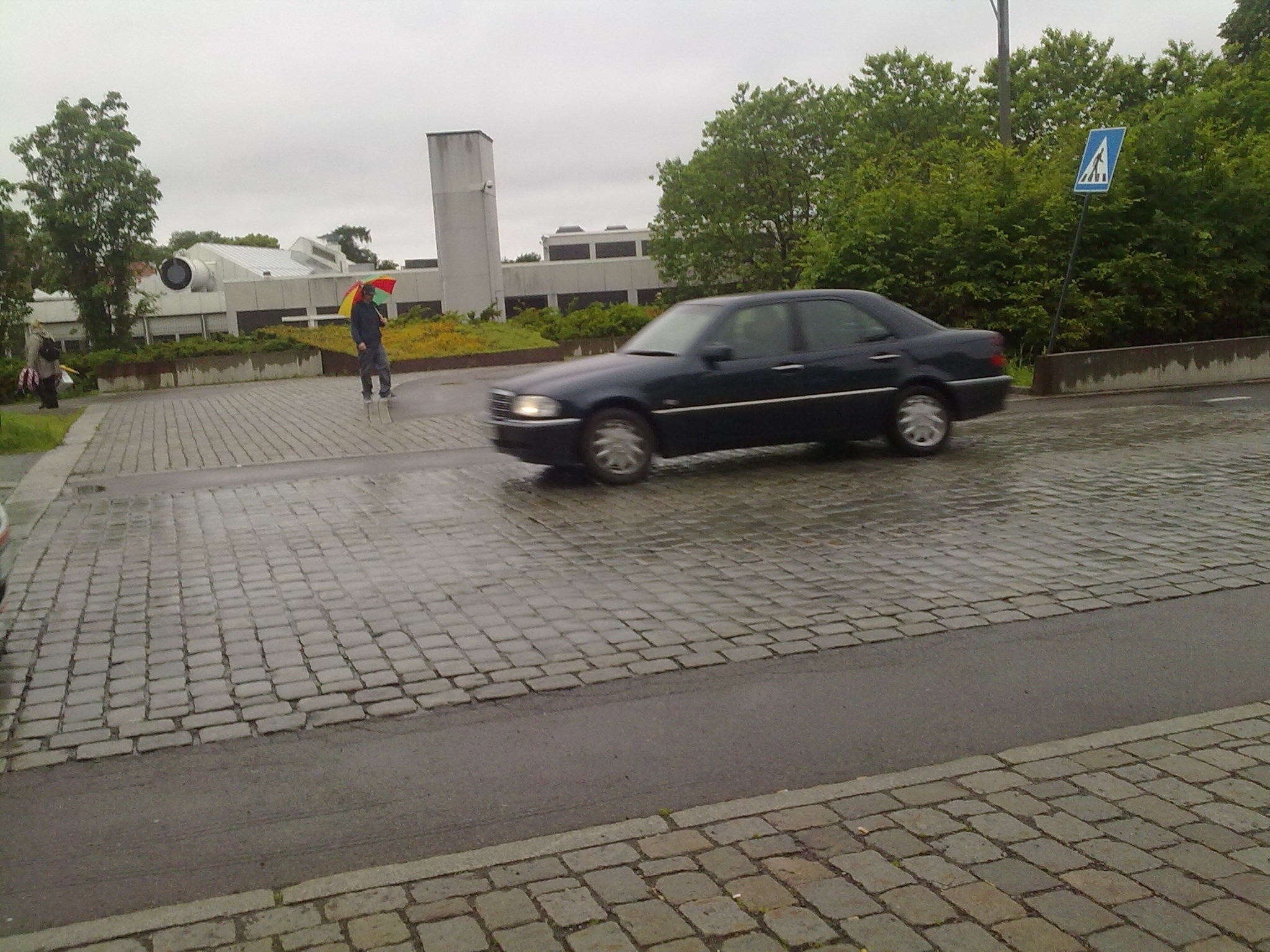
[27,319,60,409]
[350,283,397,404]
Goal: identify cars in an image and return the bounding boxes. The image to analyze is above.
[482,287,1016,487]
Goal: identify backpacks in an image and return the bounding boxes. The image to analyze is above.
[33,332,60,361]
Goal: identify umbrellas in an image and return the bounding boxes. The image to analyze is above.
[338,275,397,328]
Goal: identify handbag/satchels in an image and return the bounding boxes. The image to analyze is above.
[19,367,39,391]
[55,367,74,394]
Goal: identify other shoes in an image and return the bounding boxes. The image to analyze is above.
[363,395,374,403]
[380,390,397,401]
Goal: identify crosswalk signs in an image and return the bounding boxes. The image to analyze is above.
[1073,127,1128,194]
